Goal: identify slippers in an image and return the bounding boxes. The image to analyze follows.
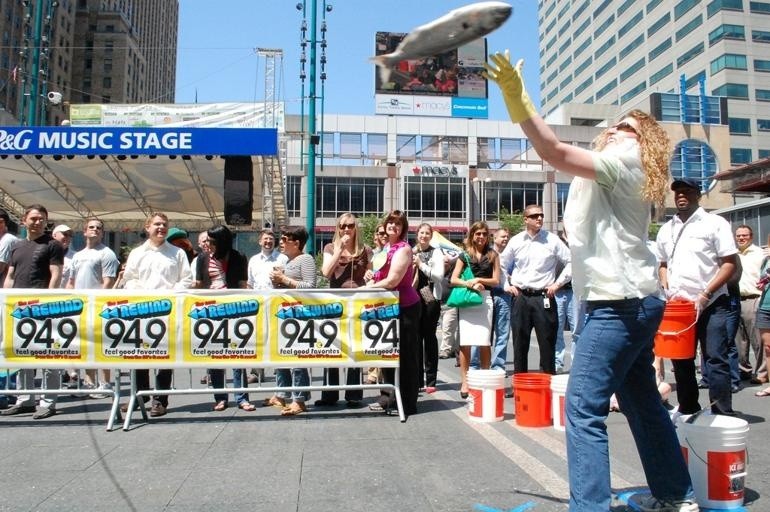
[213,401,228,410]
[281,405,305,415]
[239,402,256,411]
[263,397,285,407]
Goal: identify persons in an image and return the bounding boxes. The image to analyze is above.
[484,50,699,512]
[645,177,770,423]
[438,202,580,401]
[243,210,444,415]
[0,203,257,418]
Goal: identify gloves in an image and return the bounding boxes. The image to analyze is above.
[480,49,538,124]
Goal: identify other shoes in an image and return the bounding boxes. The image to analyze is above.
[247,374,264,384]
[120,403,138,412]
[369,396,396,410]
[315,400,337,406]
[33,406,56,419]
[90,384,113,398]
[1,405,36,416]
[346,400,363,409]
[150,403,167,416]
[71,383,95,398]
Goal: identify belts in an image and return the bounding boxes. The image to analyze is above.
[740,294,760,301]
[519,289,550,297]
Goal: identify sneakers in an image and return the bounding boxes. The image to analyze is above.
[628,491,700,512]
[696,365,769,398]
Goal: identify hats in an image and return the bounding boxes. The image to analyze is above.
[671,179,701,191]
[53,225,71,234]
[165,228,187,240]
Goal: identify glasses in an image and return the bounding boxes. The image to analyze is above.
[89,226,101,229]
[476,232,489,237]
[281,236,292,243]
[526,214,544,220]
[338,224,355,229]
[612,122,641,139]
[377,232,385,236]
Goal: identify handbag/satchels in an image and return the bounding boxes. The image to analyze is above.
[447,266,483,308]
[420,286,435,305]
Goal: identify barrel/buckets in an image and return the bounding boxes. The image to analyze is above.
[465,367,508,424]
[675,412,750,510]
[549,372,570,428]
[511,371,552,430]
[654,297,701,361]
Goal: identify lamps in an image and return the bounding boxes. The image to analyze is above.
[99,155,108,160]
[53,155,63,161]
[182,155,191,160]
[148,155,157,159]
[169,155,177,159]
[66,155,76,160]
[205,155,213,161]
[35,155,43,159]
[130,155,138,159]
[116,155,127,161]
[87,155,95,159]
[1,155,8,160]
[14,155,23,160]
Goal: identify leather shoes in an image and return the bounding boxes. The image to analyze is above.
[426,350,513,398]
